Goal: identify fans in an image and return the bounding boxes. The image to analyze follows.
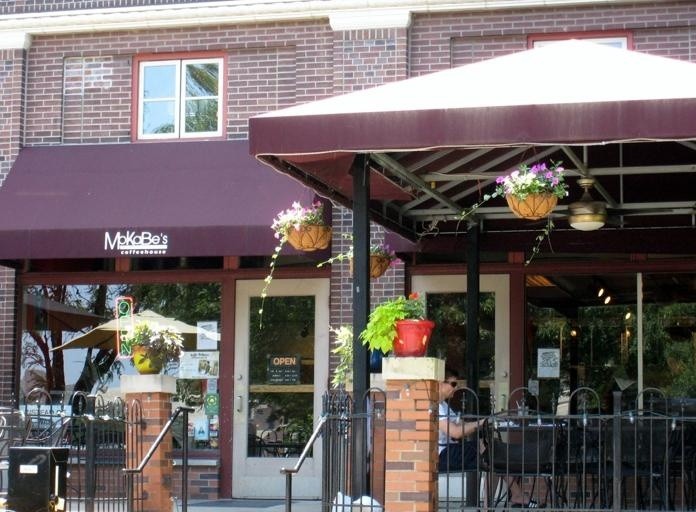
[552,147,673,214]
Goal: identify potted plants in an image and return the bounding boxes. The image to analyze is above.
[120,322,184,375]
[328,324,353,392]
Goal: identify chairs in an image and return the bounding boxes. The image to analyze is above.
[438,409,696,512]
[0,408,72,491]
[248,429,312,457]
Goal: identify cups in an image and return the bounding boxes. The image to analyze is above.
[517,406,529,419]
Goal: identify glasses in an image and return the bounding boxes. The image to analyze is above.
[443,380,457,388]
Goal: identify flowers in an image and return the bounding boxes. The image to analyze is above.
[355,291,424,356]
[317,234,401,268]
[259,200,329,330]
[456,159,570,266]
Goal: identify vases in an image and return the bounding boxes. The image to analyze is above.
[394,320,435,357]
[346,257,389,278]
[287,226,332,251]
[505,192,557,220]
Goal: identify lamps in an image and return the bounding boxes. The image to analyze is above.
[568,214,607,232]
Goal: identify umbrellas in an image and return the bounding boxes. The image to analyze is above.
[22,293,105,332]
[49,308,222,354]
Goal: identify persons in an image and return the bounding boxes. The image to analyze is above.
[439,367,547,512]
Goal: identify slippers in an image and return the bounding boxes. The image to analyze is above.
[511,497,546,509]
